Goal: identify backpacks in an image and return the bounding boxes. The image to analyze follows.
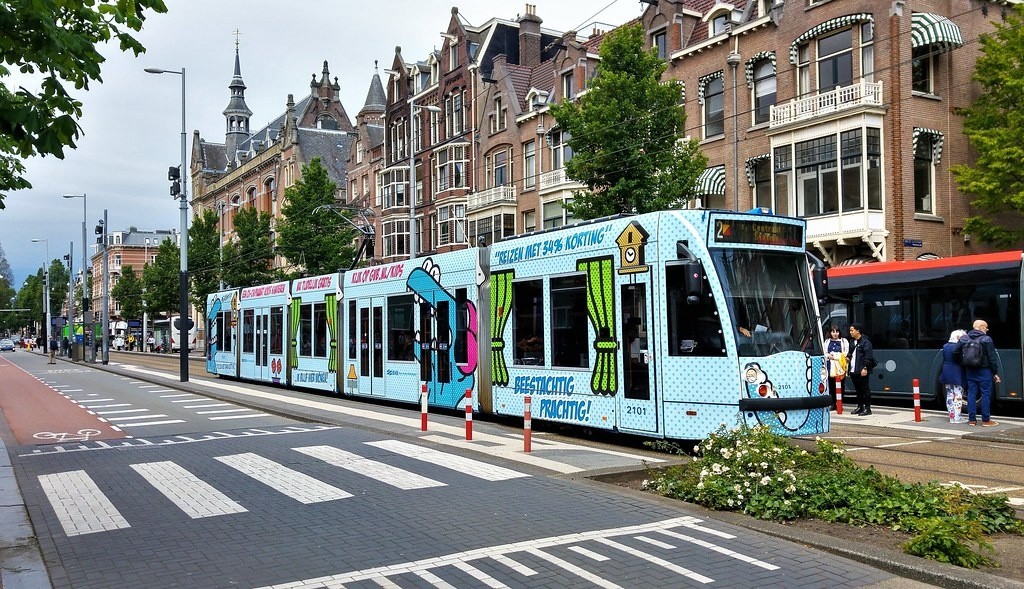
[962,335,985,366]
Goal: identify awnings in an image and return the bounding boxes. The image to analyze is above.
[689,167,725,200]
[911,127,944,165]
[911,12,962,53]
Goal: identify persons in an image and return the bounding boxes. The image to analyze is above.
[317,335,326,356]
[63,336,69,356]
[301,334,310,356]
[47,336,59,364]
[393,331,413,360]
[116,332,134,351]
[148,335,155,353]
[36,336,42,350]
[349,335,356,355]
[824,325,849,411]
[517,333,543,365]
[846,323,874,416]
[95,339,100,356]
[683,288,752,352]
[938,319,1001,427]
[19,335,26,348]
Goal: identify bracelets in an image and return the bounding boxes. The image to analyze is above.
[864,366,867,370]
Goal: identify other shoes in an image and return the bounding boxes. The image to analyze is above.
[968,420,976,425]
[830,404,836,410]
[982,421,999,427]
[950,417,969,424]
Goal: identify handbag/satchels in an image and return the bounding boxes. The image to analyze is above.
[870,358,878,368]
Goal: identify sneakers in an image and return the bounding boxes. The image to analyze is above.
[859,404,872,415]
[851,404,863,414]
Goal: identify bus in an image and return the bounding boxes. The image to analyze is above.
[810,249,1024,415]
[204,204,830,441]
[166,316,196,353]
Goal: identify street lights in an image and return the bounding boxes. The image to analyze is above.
[162,276,171,354]
[32,239,51,353]
[64,242,74,358]
[219,201,241,291]
[143,67,189,383]
[63,193,87,360]
[94,209,109,366]
[409,99,443,260]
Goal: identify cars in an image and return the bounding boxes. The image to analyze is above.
[0,335,37,352]
